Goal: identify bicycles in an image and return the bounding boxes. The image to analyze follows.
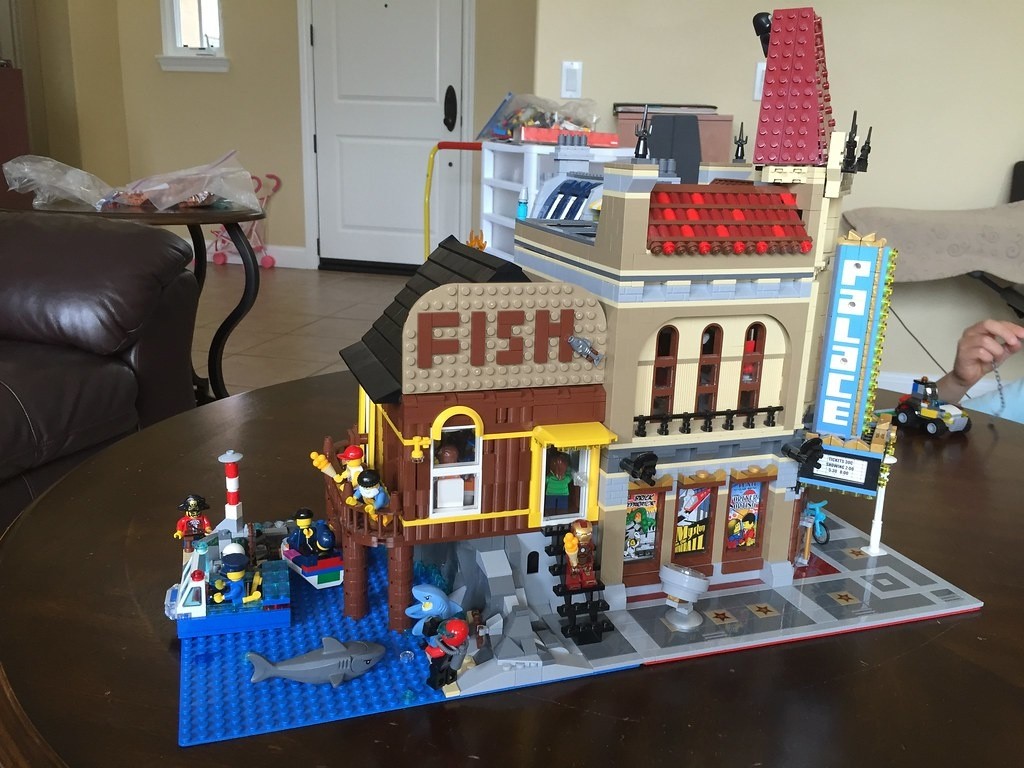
[806,500,830,545]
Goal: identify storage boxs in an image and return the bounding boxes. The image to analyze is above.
[513,125,618,147]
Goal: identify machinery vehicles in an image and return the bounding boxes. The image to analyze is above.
[894,376,971,438]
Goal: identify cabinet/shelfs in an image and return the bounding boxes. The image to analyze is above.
[481,138,634,263]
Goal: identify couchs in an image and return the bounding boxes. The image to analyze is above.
[0,210,198,533]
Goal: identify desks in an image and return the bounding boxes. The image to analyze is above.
[0,372,1024,768]
[33,194,266,406]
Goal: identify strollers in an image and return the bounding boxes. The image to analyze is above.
[192,170,281,268]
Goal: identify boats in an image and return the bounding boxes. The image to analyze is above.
[280,537,344,589]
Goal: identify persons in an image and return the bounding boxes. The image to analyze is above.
[563,519,597,590]
[433,437,475,506]
[174,495,212,548]
[545,458,572,510]
[212,544,247,604]
[288,508,335,559]
[425,619,469,690]
[935,319,1024,425]
[347,470,394,526]
[334,445,364,490]
